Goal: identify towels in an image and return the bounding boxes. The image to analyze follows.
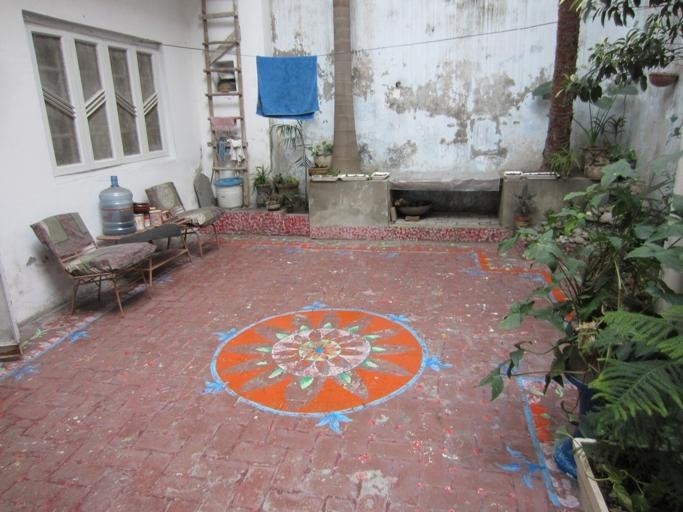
[211,115,237,134]
[213,139,227,166]
[226,138,246,166]
[253,54,319,121]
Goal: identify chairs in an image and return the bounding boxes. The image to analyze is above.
[29,211,157,318]
[144,181,226,258]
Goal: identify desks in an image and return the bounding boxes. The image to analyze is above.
[94,220,196,289]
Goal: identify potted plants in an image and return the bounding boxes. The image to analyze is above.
[565,298,683,511]
[476,157,683,480]
[274,173,301,198]
[533,33,640,182]
[308,140,334,169]
[250,161,273,207]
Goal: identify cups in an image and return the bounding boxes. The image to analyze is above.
[133,204,162,233]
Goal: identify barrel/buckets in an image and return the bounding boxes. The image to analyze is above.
[214,176,244,210]
[98,175,137,236]
